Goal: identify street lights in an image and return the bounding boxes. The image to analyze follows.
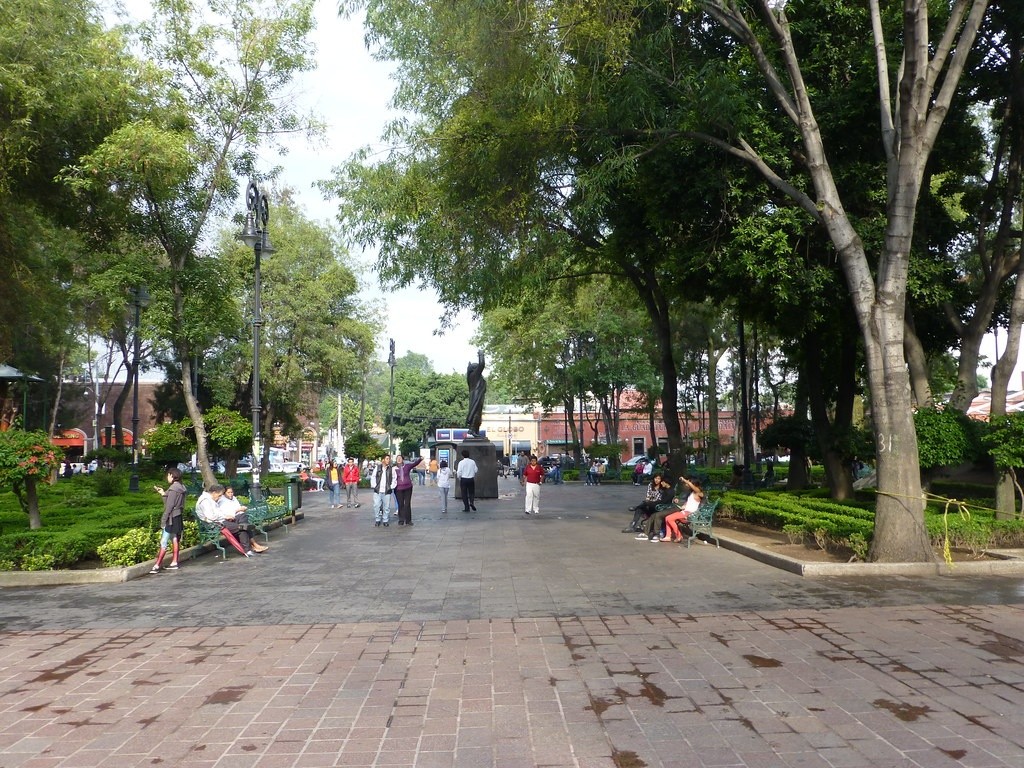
[128,285,150,493]
[386,337,398,461]
[238,181,279,507]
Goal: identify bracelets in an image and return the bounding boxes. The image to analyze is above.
[540,482,542,484]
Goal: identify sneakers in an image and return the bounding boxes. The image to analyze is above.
[163,564,179,569]
[150,567,160,574]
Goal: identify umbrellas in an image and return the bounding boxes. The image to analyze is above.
[214,519,249,557]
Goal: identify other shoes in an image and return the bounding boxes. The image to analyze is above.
[394,512,398,515]
[660,537,671,541]
[462,509,469,512]
[470,504,476,511]
[246,525,254,531]
[384,522,389,526]
[622,527,633,533]
[651,536,659,543]
[442,509,446,512]
[406,522,412,525]
[355,504,358,508]
[535,511,539,514]
[674,537,682,542]
[525,511,530,514]
[398,521,404,525]
[254,546,267,553]
[375,522,380,526]
[635,533,648,540]
[337,505,343,508]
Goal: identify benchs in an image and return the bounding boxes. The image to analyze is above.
[655,496,721,549]
[189,502,268,559]
[246,499,288,541]
[293,474,325,491]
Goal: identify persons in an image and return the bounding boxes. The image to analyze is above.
[416,457,453,512]
[585,461,605,486]
[196,484,269,555]
[621,473,706,542]
[150,469,187,573]
[661,453,671,476]
[544,453,571,481]
[631,459,652,485]
[63,461,80,477]
[457,451,478,511]
[299,458,361,508]
[523,456,545,514]
[371,455,424,526]
[501,452,536,482]
[467,350,486,437]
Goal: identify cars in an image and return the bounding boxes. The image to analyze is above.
[508,452,608,475]
[196,460,305,473]
[622,455,656,466]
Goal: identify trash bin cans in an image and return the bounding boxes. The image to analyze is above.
[283,474,303,510]
